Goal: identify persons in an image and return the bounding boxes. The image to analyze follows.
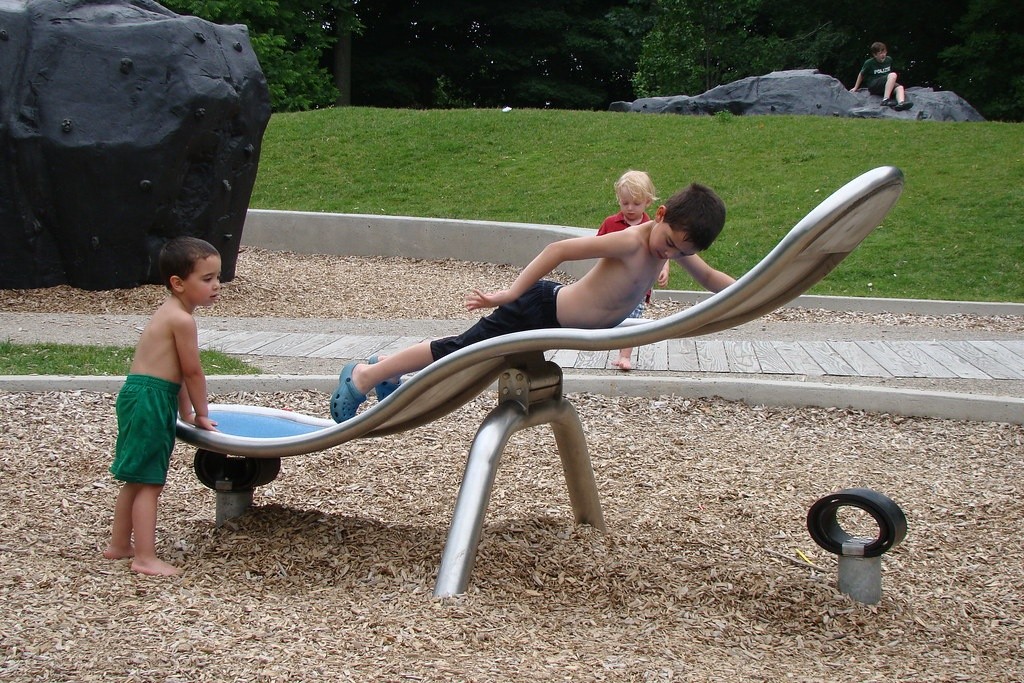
[330,183,736,424]
[596,171,669,370]
[104,236,221,577]
[850,41,913,111]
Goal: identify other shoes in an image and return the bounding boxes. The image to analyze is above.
[881,97,897,106]
[894,101,913,111]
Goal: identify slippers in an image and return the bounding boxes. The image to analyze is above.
[369,355,403,404]
[328,362,367,425]
